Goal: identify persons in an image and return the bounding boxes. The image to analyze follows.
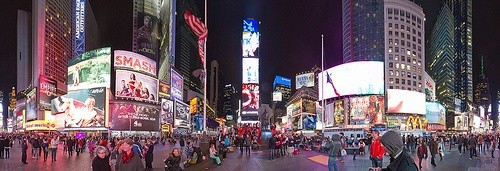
[134,14,160,56]
[52,64,103,127]
[0,122,500,171]
[363,95,383,126]
[115,73,157,101]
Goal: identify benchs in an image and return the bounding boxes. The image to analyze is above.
[300,145,366,155]
[182,154,223,171]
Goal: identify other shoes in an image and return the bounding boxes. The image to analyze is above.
[218,163,222,166]
[431,161,436,166]
[469,157,480,161]
[268,154,289,160]
[419,165,422,171]
[340,160,344,162]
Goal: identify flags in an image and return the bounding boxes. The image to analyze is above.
[184,10,208,69]
